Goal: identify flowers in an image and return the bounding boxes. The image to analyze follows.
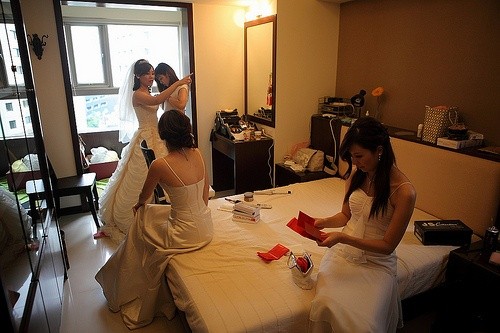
[371,86,384,118]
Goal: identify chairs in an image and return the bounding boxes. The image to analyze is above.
[26,179,45,241]
[46,152,101,230]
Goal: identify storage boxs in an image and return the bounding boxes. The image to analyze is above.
[4,167,43,192]
[438,135,486,149]
[413,219,474,248]
[84,153,118,182]
[232,201,260,223]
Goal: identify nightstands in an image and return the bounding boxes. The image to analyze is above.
[275,162,340,189]
[430,239,500,333]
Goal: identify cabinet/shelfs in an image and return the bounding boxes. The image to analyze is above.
[0,0,68,333]
[211,132,274,195]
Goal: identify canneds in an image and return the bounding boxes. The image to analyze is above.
[250,131,255,141]
[483,226,498,255]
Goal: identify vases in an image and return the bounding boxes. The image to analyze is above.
[371,96,384,122]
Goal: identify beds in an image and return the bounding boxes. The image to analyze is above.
[161,125,500,333]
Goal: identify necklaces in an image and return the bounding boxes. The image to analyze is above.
[366,172,374,182]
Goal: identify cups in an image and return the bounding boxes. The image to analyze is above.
[255,131,262,140]
[242,131,251,142]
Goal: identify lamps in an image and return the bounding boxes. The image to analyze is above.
[351,90,366,119]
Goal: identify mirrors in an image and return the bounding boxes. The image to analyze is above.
[244,13,277,129]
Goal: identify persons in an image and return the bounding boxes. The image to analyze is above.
[95,110,215,330]
[154,63,189,113]
[0,185,32,255]
[97,59,194,248]
[307,116,416,333]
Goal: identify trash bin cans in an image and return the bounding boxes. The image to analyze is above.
[60,229,70,271]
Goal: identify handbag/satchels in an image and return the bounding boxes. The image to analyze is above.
[292,148,324,172]
[422,104,460,144]
[287,251,314,290]
